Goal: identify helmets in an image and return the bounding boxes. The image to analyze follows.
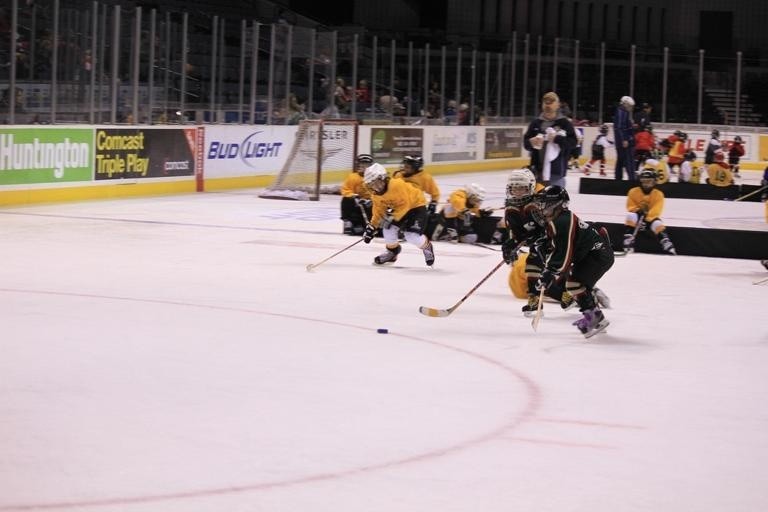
[401,153,423,177]
[600,125,608,133]
[735,136,741,143]
[715,152,724,161]
[685,152,695,160]
[363,163,387,196]
[639,168,656,194]
[466,183,487,208]
[505,168,536,206]
[534,185,569,222]
[712,130,719,137]
[675,130,687,143]
[355,154,373,175]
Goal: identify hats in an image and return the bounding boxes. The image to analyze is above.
[620,96,635,106]
[543,91,559,103]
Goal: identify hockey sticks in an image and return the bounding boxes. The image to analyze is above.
[420,241,528,318]
[613,214,646,257]
[306,230,379,272]
[531,285,545,330]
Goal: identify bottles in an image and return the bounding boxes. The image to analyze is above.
[533,134,544,151]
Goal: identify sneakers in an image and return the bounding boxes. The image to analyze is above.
[397,229,405,239]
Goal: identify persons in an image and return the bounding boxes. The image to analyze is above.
[338,153,385,240]
[361,161,438,266]
[756,163,768,272]
[620,167,677,252]
[534,185,614,335]
[498,166,573,313]
[2,0,502,124]
[521,91,746,203]
[392,151,441,219]
[431,181,487,245]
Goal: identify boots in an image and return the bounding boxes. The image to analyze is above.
[599,166,606,175]
[344,220,353,233]
[561,292,575,309]
[523,293,543,311]
[374,242,401,264]
[582,165,590,176]
[733,170,742,178]
[576,305,605,333]
[594,288,609,307]
[621,234,636,248]
[566,160,579,170]
[660,237,673,250]
[422,239,434,266]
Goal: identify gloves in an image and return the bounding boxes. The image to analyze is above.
[363,223,375,244]
[378,213,394,229]
[353,194,372,210]
[484,206,493,213]
[428,200,437,214]
[535,269,555,291]
[502,241,519,265]
